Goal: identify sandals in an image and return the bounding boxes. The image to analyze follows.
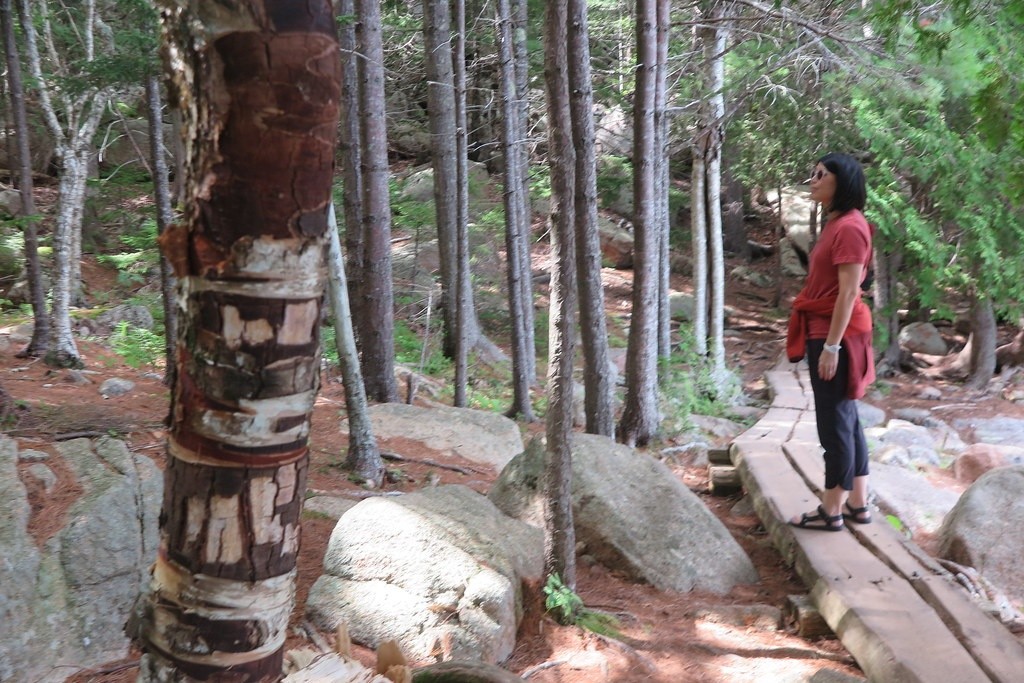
[790,505,843,530]
[842,499,872,523]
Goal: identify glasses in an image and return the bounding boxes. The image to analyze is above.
[810,170,836,180]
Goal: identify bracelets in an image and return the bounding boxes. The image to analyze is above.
[823,343,841,353]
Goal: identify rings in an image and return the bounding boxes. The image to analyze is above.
[828,371,832,375]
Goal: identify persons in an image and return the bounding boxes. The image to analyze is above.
[785,157,877,532]
